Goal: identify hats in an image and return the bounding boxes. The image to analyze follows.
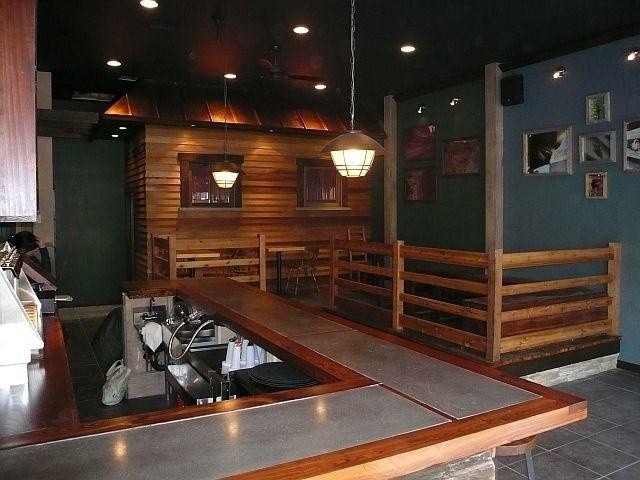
[12,231,39,251]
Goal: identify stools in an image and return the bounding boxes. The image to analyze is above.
[495,435,535,480]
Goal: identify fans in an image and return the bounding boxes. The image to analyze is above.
[234,43,328,84]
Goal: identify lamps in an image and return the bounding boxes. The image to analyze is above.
[554,66,565,80]
[207,56,246,189]
[449,96,461,107]
[418,105,425,112]
[627,47,640,63]
[319,0,388,178]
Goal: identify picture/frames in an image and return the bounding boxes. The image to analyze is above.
[585,91,611,127]
[521,125,572,177]
[585,171,608,200]
[622,117,640,177]
[579,130,617,165]
[402,122,439,161]
[403,164,440,203]
[440,136,484,177]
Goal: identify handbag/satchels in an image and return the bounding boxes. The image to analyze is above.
[102,359,131,406]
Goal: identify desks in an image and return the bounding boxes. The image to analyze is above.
[265,245,306,296]
[178,251,219,278]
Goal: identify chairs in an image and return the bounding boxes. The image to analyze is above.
[285,245,320,294]
[348,226,380,282]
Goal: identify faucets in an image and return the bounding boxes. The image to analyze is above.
[149,297,156,313]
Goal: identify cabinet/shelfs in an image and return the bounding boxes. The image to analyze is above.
[1,0,37,225]
